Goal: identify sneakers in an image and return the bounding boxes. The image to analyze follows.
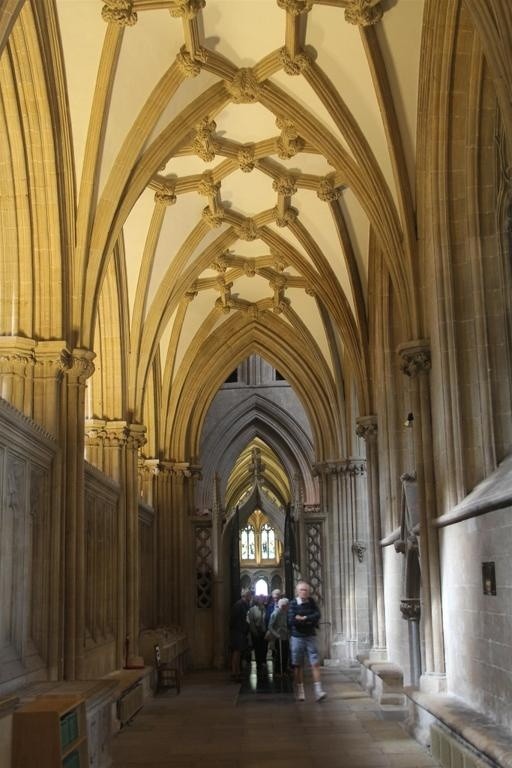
[299,694,304,700]
[315,692,327,702]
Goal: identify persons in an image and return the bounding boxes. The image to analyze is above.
[287,582,327,704]
[229,588,295,696]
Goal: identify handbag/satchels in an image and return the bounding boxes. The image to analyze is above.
[264,624,278,640]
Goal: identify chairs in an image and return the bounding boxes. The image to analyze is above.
[154,643,180,695]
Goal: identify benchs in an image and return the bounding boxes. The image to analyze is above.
[97,663,155,731]
[354,653,512,767]
